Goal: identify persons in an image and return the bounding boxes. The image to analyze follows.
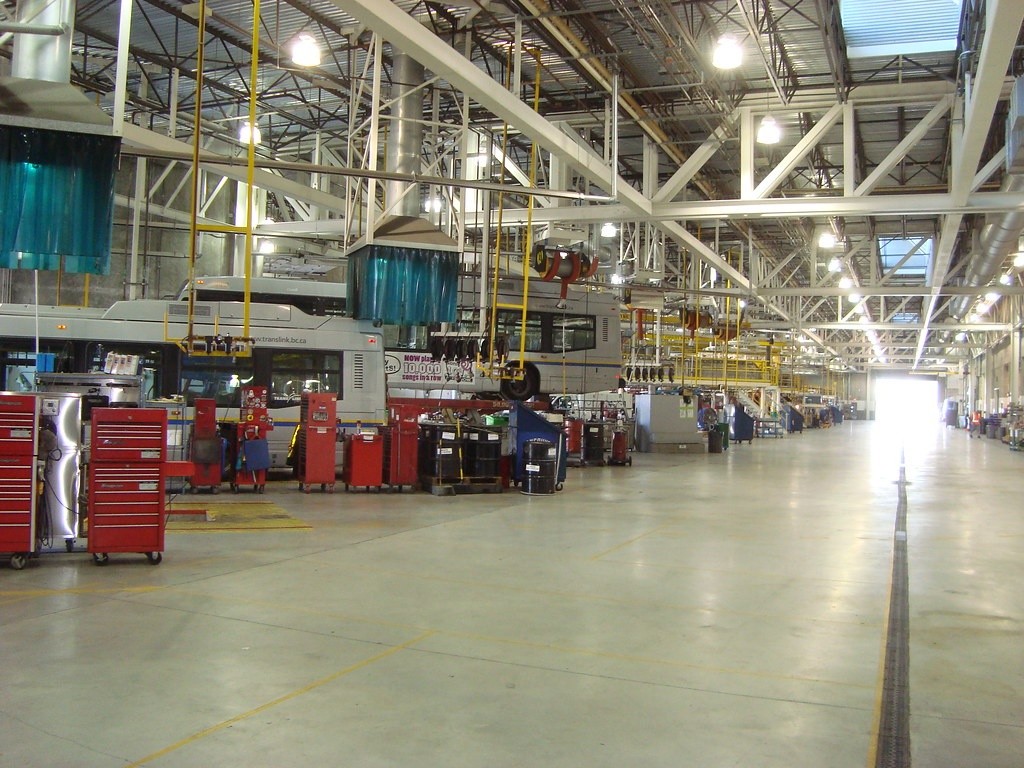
[969,409,982,438]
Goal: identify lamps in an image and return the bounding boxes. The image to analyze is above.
[291,0,322,68]
[239,101,261,146]
[600,221,618,238]
[712,0,744,70]
[818,216,886,364]
[956,253,1024,341]
[756,74,782,144]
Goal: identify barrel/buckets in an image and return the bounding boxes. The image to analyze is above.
[716,423,729,447]
[563,419,584,453]
[418,414,512,488]
[709,430,723,453]
[521,442,555,496]
[581,423,604,460]
[610,430,628,460]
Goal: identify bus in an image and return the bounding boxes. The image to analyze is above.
[1,295,392,480]
[171,273,627,406]
